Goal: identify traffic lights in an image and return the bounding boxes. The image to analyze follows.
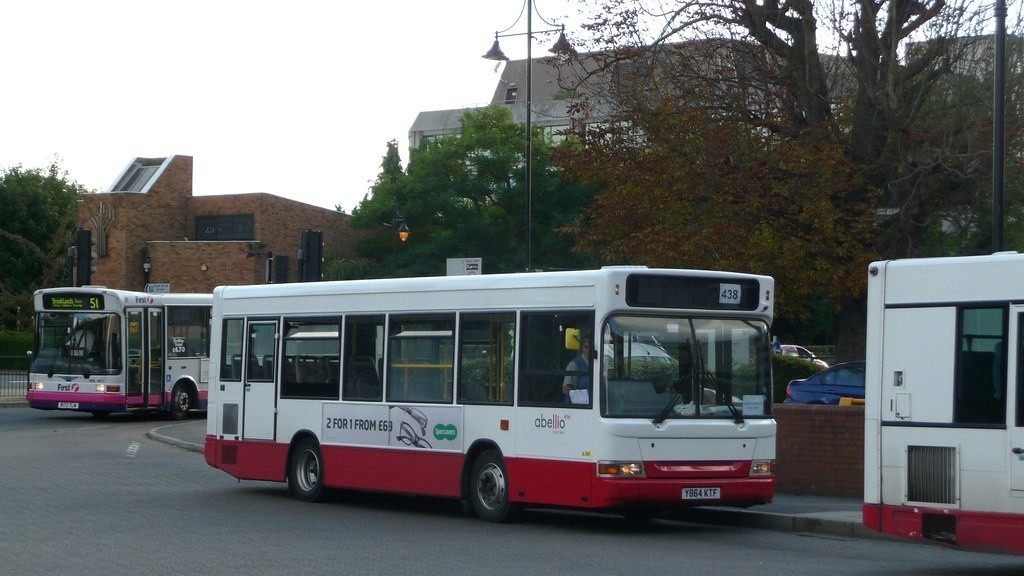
[90,238,98,273]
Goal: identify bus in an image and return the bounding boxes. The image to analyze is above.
[862,250,1024,557]
[26,285,338,421]
[204,265,778,524]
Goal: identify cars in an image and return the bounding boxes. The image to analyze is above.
[782,360,866,406]
[780,344,828,369]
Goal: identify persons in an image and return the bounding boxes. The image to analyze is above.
[65,321,95,361]
[562,336,607,406]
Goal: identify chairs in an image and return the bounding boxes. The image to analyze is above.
[229,353,486,403]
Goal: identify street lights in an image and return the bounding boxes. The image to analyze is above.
[480,0,578,273]
[383,196,410,277]
